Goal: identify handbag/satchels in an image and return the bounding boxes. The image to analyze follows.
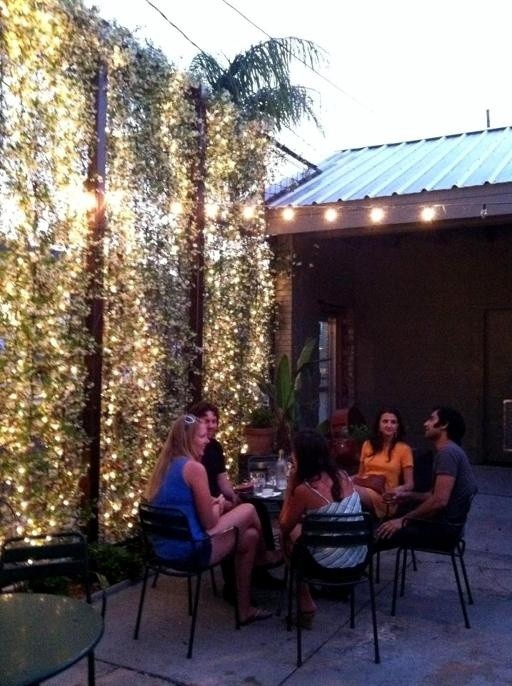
[352,473,386,495]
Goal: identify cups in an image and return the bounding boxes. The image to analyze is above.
[249,470,266,496]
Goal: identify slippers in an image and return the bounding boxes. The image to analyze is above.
[240,607,272,625]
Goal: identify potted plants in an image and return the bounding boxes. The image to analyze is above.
[242,405,281,454]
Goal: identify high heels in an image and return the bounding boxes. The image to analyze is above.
[290,611,316,630]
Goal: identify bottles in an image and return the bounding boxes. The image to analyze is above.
[274,448,288,492]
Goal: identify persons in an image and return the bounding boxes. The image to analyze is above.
[374,407,475,554]
[279,429,368,614]
[145,414,284,626]
[189,402,275,551]
[351,408,414,517]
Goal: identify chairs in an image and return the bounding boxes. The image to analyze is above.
[131,494,240,659]
[1,529,99,685]
[391,485,474,629]
[284,512,381,666]
[150,478,417,615]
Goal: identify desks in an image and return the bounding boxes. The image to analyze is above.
[1,593,105,686]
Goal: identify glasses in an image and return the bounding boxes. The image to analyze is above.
[184,414,197,432]
